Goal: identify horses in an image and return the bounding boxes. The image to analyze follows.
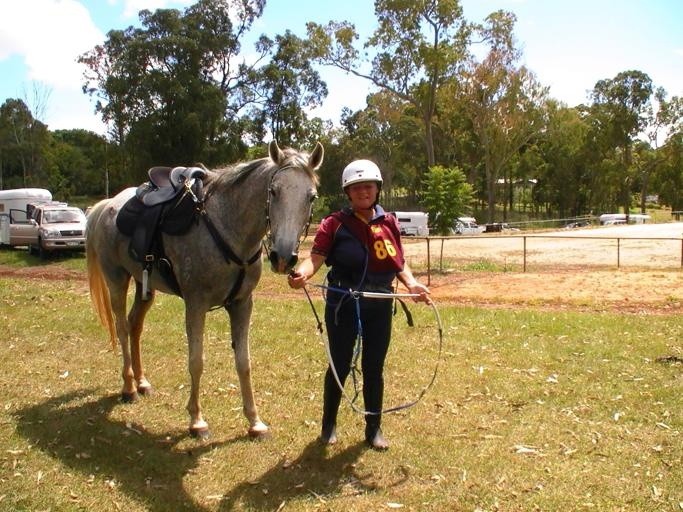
[83,140,324,441]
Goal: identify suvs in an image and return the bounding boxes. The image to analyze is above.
[451,221,484,235]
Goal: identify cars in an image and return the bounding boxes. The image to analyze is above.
[606,220,625,225]
[9,202,88,257]
[564,222,589,229]
[644,195,657,202]
[80,206,91,216]
[490,221,519,232]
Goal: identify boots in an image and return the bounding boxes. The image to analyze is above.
[318,365,351,446]
[361,380,390,451]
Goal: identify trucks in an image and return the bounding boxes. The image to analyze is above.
[386,211,431,238]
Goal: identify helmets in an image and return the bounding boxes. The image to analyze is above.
[339,158,384,190]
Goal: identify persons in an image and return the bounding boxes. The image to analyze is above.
[287,159,432,451]
